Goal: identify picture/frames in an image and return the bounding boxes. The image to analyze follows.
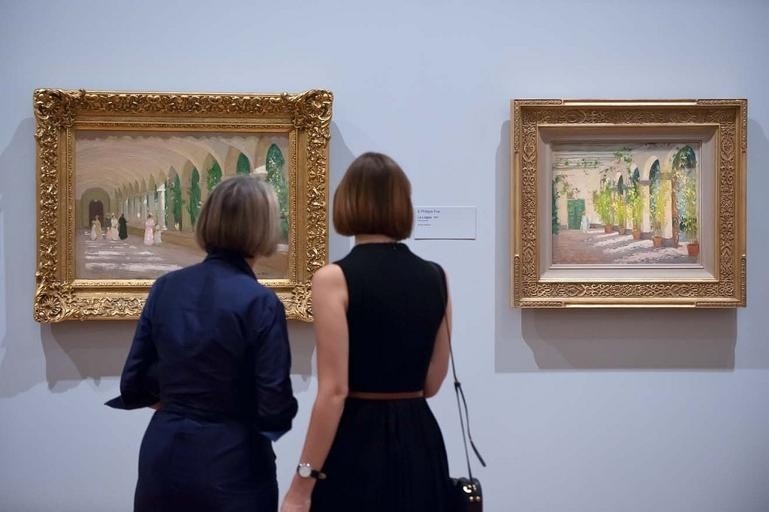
[33,89,334,325]
[509,98,747,307]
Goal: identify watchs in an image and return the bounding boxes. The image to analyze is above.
[294,462,327,481]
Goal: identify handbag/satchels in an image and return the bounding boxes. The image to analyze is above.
[446,477,483,512]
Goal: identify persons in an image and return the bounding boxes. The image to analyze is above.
[118,214,129,239]
[91,214,103,241]
[144,214,156,245]
[580,211,590,233]
[110,212,119,240]
[105,226,111,240]
[105,215,111,227]
[103,175,298,512]
[153,224,162,246]
[278,151,453,512]
